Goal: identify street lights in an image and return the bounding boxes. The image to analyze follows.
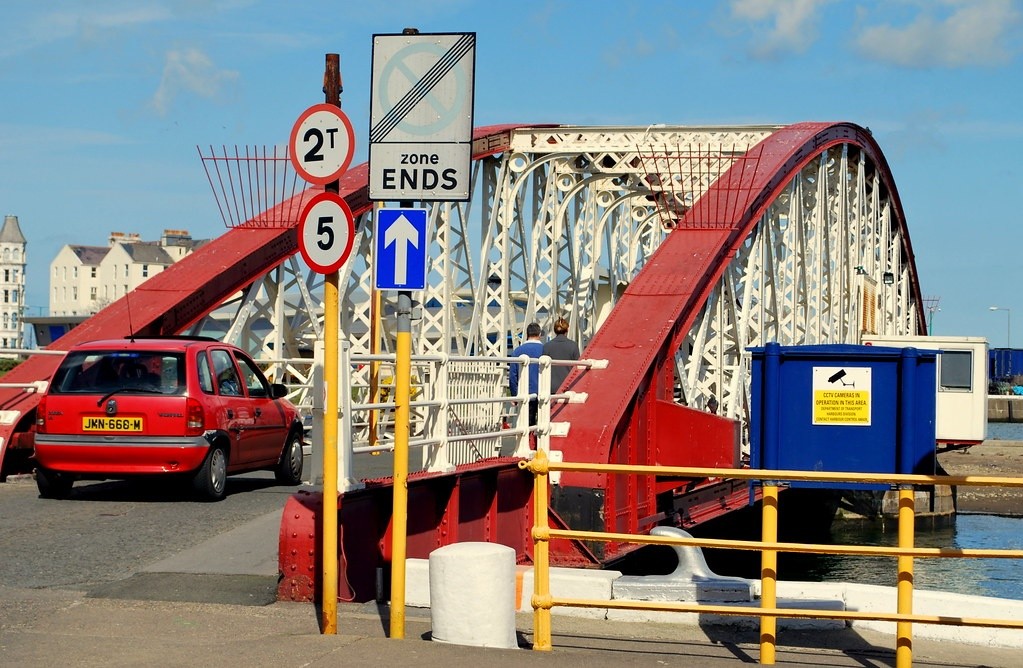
[927,305,941,336]
[989,307,1011,348]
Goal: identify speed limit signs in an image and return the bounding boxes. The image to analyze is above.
[297,192,356,274]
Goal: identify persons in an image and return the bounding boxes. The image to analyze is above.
[544,318,580,405]
[508,323,545,427]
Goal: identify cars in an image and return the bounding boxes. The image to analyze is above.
[29,336,305,502]
